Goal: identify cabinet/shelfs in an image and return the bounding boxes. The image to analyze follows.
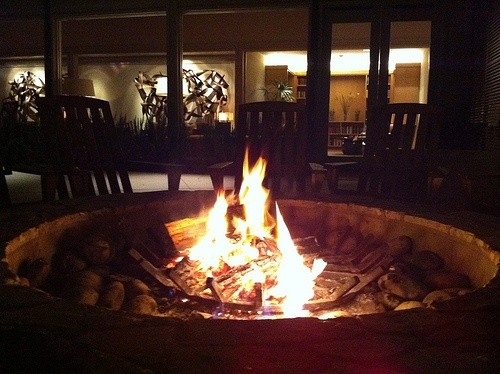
[264,67,306,103]
[327,121,364,149]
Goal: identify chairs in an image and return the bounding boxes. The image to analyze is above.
[35,95,137,196]
[235,102,313,194]
[358,102,445,205]
